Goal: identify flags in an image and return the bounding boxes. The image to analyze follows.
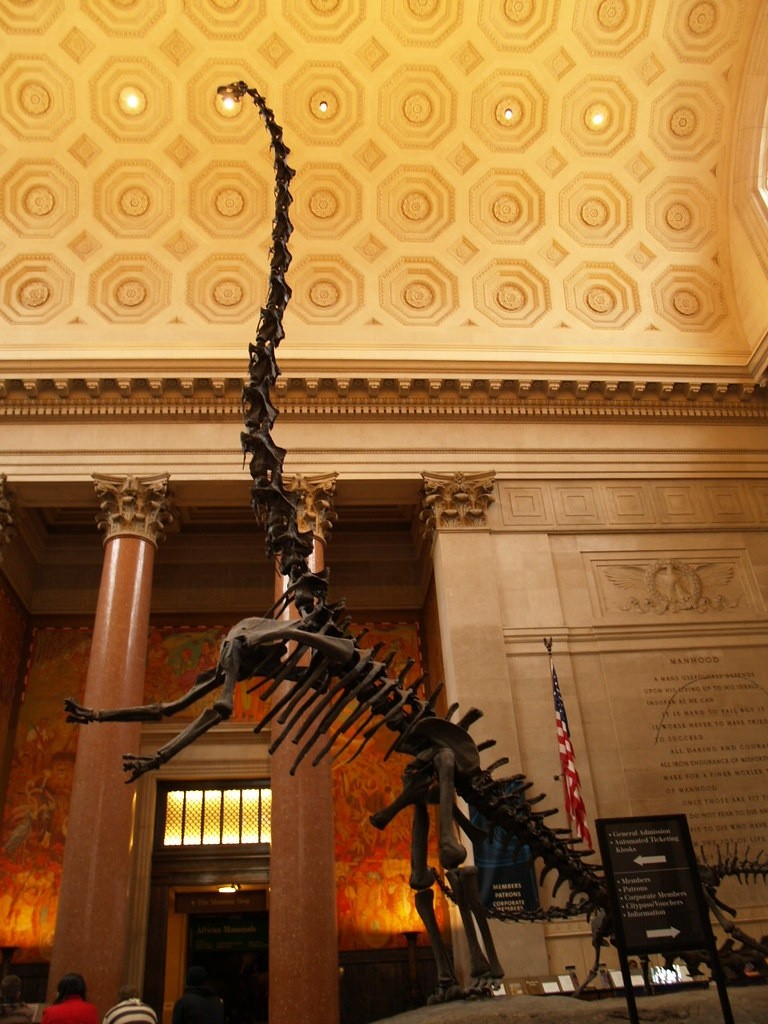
[549,661,592,851]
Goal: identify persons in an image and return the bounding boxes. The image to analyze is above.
[0,974,34,1024]
[39,973,99,1024]
[173,939,267,1024]
[427,980,450,1006]
[744,935,768,977]
[101,985,158,1024]
[401,987,422,1011]
